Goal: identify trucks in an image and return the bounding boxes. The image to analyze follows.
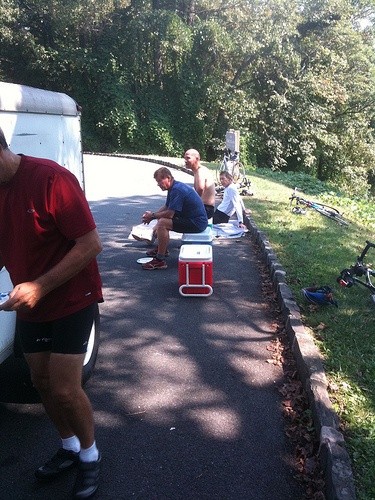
[0,81,101,386]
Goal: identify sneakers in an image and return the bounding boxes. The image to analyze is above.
[142,257,167,270]
[72,455,102,500]
[35,448,80,479]
[146,246,169,257]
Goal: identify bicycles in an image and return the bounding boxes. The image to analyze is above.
[288,187,350,227]
[337,240,375,306]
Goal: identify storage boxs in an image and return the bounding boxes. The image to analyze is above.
[178,244,213,296]
[180,226,213,247]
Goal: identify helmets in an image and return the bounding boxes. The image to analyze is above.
[302,285,333,305]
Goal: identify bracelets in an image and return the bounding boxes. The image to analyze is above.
[153,213,156,219]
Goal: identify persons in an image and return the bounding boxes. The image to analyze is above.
[146,149,215,245]
[141,167,208,270]
[213,169,252,230]
[0,131,104,499]
